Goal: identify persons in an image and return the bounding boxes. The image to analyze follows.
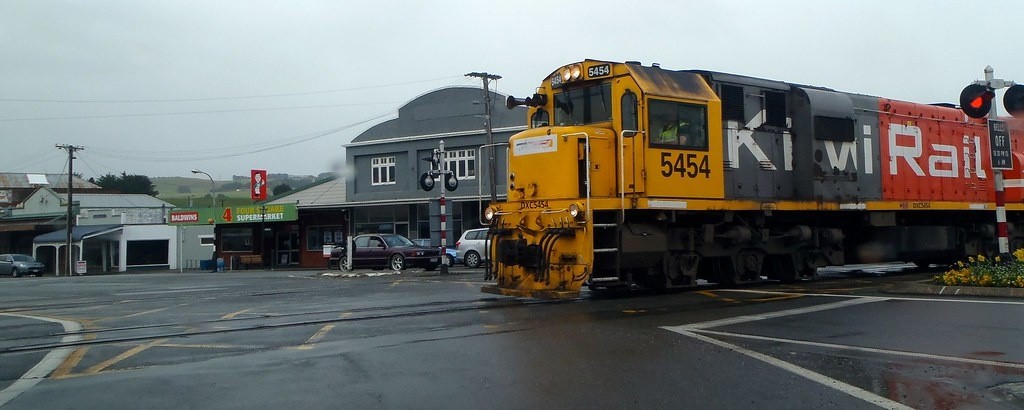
[655,108,690,146]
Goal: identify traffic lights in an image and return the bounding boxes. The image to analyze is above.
[960,84,995,119]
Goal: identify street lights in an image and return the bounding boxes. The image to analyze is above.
[191,169,216,221]
[474,99,496,203]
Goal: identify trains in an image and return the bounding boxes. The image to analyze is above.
[481,57,1024,301]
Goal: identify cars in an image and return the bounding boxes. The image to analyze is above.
[328,232,442,272]
[0,254,47,278]
[405,238,463,268]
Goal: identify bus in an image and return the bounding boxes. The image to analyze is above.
[455,227,493,269]
